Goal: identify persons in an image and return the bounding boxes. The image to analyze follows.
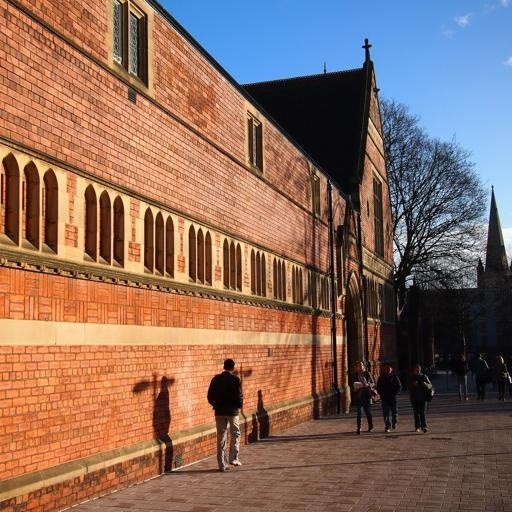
[376,364,403,433]
[350,360,376,435]
[408,364,434,434]
[492,354,509,402]
[206,358,246,472]
[453,353,470,401]
[472,353,489,401]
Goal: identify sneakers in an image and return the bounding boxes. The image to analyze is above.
[415,428,427,433]
[220,460,242,471]
[357,428,372,435]
[384,429,390,432]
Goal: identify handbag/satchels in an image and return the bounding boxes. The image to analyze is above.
[427,388,435,402]
